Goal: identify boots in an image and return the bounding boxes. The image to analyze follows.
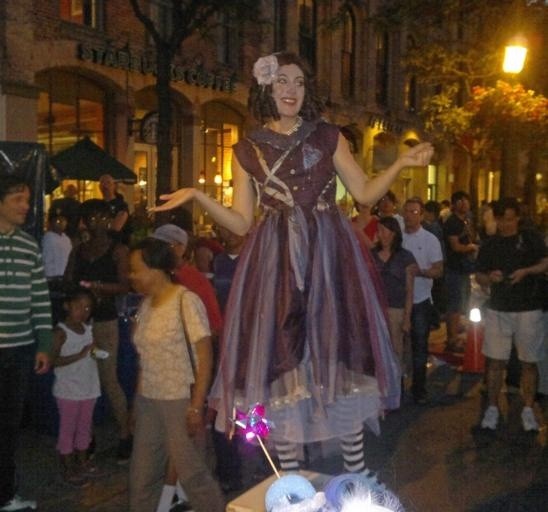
[59,451,104,488]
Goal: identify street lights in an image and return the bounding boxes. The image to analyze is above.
[503,0,547,78]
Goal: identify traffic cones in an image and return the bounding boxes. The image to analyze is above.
[455,296,491,383]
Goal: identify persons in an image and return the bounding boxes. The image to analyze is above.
[2,175,271,512]
[355,191,547,439]
[146,51,434,490]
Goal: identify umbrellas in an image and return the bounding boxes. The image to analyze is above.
[45,136,138,203]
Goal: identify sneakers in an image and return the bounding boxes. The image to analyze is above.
[522,407,537,432]
[480,406,501,431]
[0,496,37,512]
[117,436,133,466]
